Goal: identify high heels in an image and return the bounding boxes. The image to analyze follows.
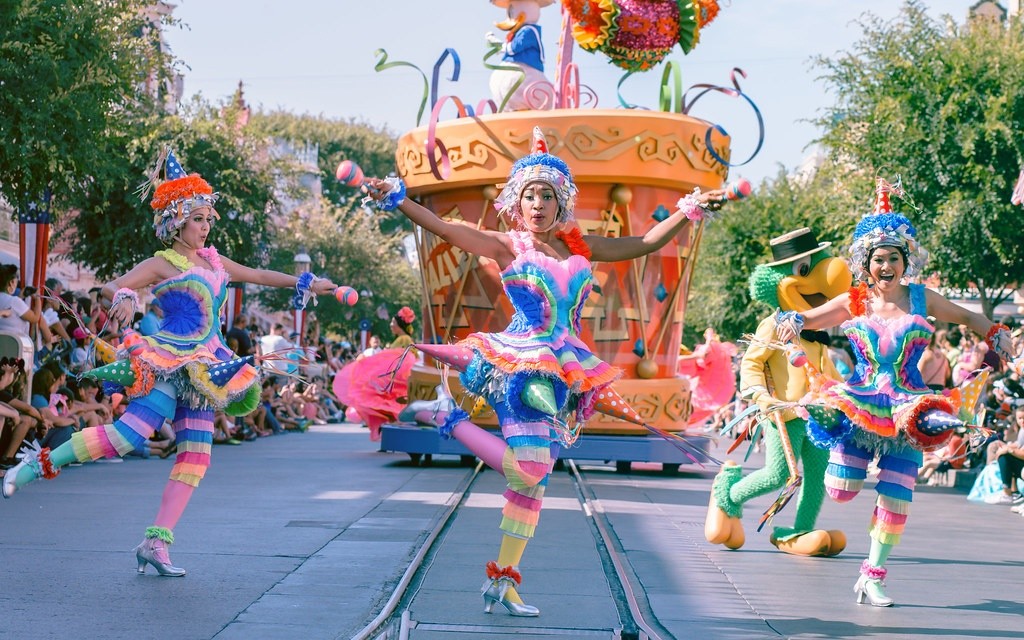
[479,560,540,617]
[136,526,186,576]
[2,438,61,499]
[397,384,470,441]
[852,559,894,607]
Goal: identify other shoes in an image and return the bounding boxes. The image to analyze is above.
[300,418,313,432]
[159,443,177,459]
[1011,491,1024,505]
[95,455,124,463]
[983,489,1012,505]
[0,456,23,471]
[161,436,176,453]
[915,477,928,484]
[225,437,242,445]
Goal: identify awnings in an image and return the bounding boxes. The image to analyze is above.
[915,286,1024,320]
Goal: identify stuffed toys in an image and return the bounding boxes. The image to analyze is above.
[704,229,859,556]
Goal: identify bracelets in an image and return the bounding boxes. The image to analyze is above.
[677,188,710,223]
[778,310,804,336]
[112,288,138,323]
[376,176,407,210]
[293,272,318,310]
[985,324,1010,354]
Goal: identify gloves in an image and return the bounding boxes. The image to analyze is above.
[753,390,784,417]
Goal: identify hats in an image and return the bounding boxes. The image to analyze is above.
[848,164,931,286]
[764,226,832,267]
[132,143,221,242]
[494,125,579,233]
[8,356,26,374]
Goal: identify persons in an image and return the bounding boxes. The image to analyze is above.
[774,179,1014,607]
[716,315,1024,515]
[0,263,385,479]
[3,143,337,577]
[375,307,418,453]
[367,126,727,618]
